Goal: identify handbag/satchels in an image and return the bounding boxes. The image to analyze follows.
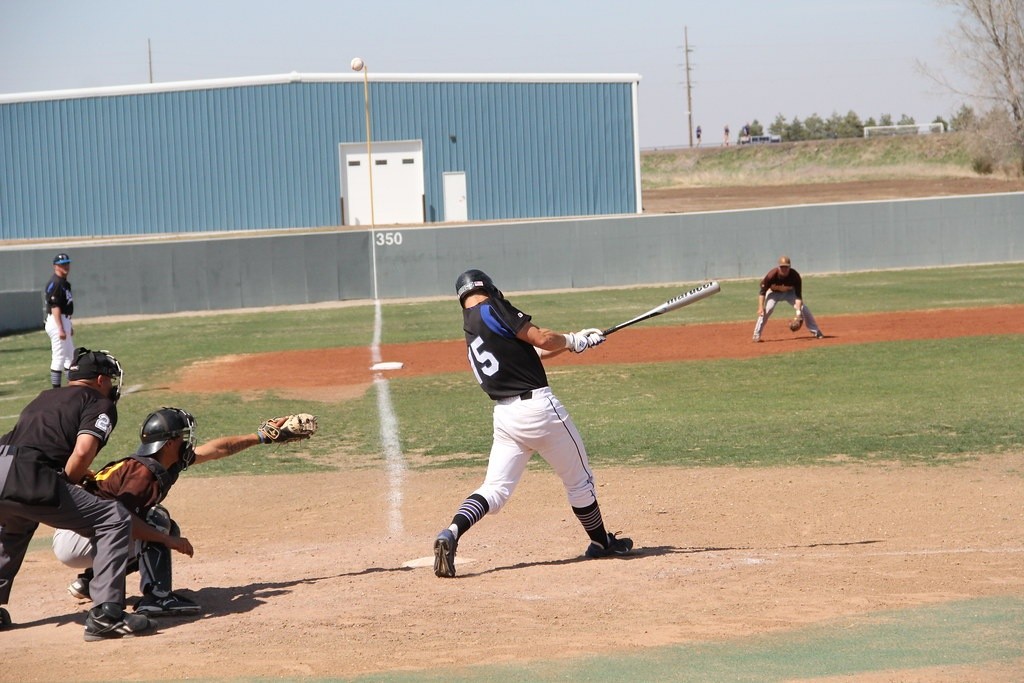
[0,448,60,506]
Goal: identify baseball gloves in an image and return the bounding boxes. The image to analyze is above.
[788,314,803,332]
[257,413,320,454]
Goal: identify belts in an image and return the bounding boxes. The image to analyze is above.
[0,445,14,456]
[49,312,71,319]
[520,391,533,401]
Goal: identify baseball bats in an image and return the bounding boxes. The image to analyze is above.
[568,279,722,352]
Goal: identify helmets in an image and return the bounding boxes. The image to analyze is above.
[136,410,187,456]
[455,269,504,308]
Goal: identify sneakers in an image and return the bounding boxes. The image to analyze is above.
[84,608,159,642]
[433,530,457,577]
[134,592,201,616]
[66,578,93,603]
[585,530,632,557]
[0,607,10,631]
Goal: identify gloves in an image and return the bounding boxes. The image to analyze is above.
[568,328,606,353]
[569,332,589,354]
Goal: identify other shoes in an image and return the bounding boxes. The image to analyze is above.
[815,330,824,338]
[753,336,760,342]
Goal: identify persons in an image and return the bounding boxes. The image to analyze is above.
[0,347,157,641]
[433,269,633,577]
[51,406,316,615]
[44,252,74,388]
[751,256,824,342]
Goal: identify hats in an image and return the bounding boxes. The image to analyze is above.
[778,256,790,267]
[53,253,71,265]
[68,354,104,381]
